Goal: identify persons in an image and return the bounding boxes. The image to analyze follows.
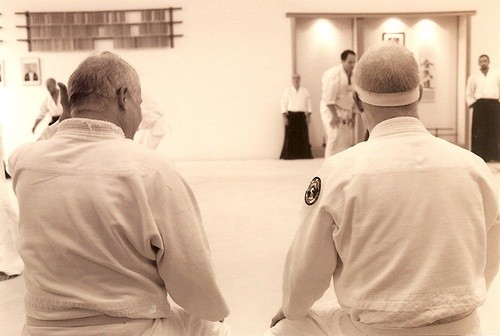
[467,54,500,163]
[32,78,63,134]
[262,40,500,336]
[279,73,313,160]
[25,63,38,80]
[320,49,358,160]
[13,50,231,336]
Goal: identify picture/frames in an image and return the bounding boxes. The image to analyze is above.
[382,32,405,46]
[20,58,42,86]
